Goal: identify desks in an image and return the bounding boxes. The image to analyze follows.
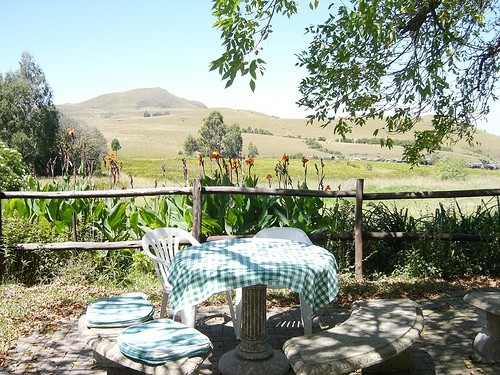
[164,237,339,375]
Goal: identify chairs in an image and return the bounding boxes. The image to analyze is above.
[141,227,235,329]
[235,227,315,339]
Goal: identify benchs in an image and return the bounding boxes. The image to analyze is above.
[79,292,215,375]
[464,292,500,364]
[283,297,425,375]
